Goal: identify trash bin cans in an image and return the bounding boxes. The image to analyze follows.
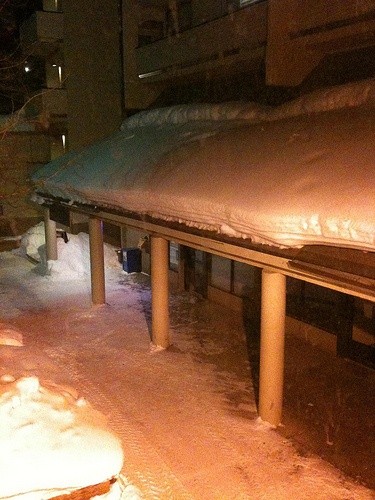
[122,248,142,273]
